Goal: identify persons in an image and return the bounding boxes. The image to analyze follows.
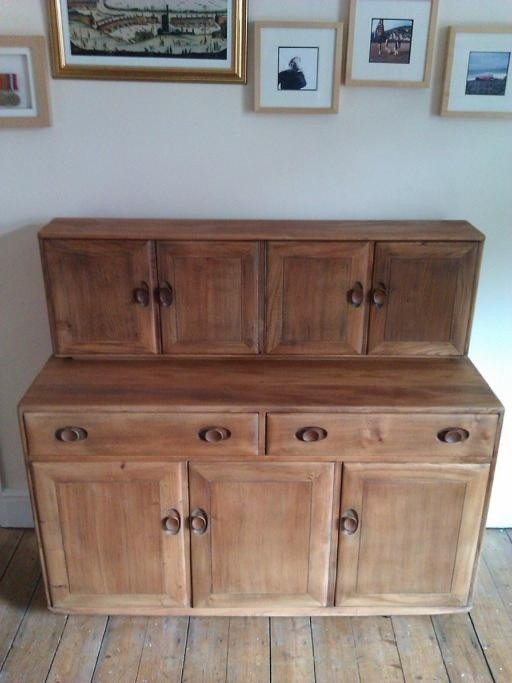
[277,55,306,89]
[374,17,404,57]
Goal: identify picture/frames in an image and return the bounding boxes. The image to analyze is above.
[252,20,343,116]
[343,0,439,89]
[0,34,54,130]
[46,1,250,85]
[440,23,512,120]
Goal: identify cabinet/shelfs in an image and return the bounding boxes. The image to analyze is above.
[17,351,505,618]
[38,215,488,361]
